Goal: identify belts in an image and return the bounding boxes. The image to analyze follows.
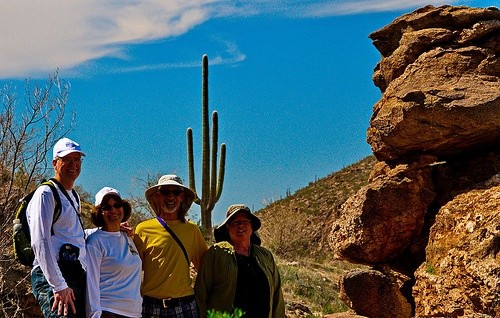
[142,294,195,308]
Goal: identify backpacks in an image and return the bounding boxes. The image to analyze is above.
[12,180,80,266]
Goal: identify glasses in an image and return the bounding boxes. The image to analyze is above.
[159,189,183,197]
[100,202,122,211]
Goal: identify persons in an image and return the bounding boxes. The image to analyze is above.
[193,204,285,318]
[132,175,209,318]
[86,186,143,318]
[26,138,133,318]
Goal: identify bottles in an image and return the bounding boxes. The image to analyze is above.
[12,218,22,233]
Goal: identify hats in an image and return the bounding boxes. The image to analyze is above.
[53,138,86,159]
[216,204,261,233]
[145,175,195,214]
[95,187,121,207]
